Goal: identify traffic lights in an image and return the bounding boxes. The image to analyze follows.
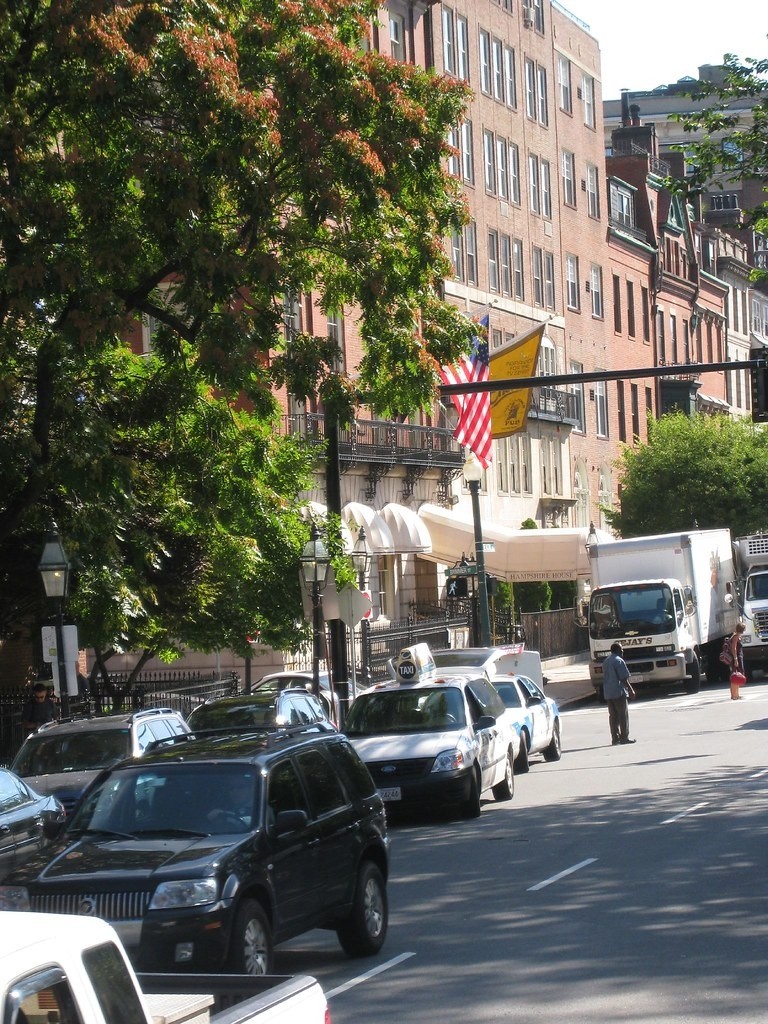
[447,578,468,597]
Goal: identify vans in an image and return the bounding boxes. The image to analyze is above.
[339,646,522,820]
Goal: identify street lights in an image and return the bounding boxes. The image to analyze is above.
[349,525,371,687]
[463,449,492,649]
[35,517,72,723]
[297,522,331,701]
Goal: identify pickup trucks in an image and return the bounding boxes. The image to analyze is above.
[0,911,330,1023]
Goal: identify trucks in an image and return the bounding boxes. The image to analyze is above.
[730,533,768,683]
[577,527,741,703]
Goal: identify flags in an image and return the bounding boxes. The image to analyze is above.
[489,323,546,440]
[431,313,493,470]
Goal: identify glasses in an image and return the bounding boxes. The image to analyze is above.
[35,696,44,699]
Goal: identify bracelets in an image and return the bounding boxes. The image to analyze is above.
[734,657,737,660]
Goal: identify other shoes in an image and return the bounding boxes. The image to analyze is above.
[731,696,741,700]
[619,739,636,744]
[612,740,620,745]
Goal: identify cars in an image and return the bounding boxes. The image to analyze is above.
[0,768,67,887]
[487,651,564,775]
[241,670,368,720]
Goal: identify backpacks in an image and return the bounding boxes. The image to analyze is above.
[719,634,736,665]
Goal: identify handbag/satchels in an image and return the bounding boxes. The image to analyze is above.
[730,668,746,686]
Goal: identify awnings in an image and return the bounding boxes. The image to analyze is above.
[379,502,433,554]
[340,501,395,557]
[301,500,354,556]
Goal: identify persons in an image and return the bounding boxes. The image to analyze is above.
[728,623,746,700]
[208,778,275,831]
[75,661,90,700]
[601,643,637,745]
[20,683,58,736]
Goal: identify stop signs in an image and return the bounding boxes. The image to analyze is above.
[359,590,373,620]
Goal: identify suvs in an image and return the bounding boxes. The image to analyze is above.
[0,723,394,1014]
[182,688,337,739]
[9,708,198,831]
